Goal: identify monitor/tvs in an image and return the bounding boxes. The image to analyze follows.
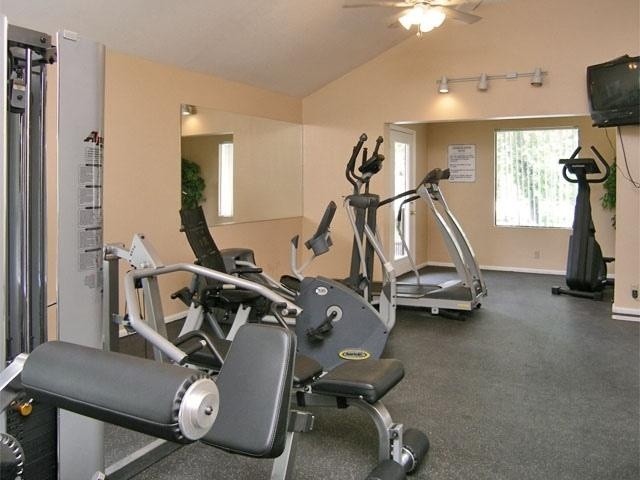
[587,54,640,127]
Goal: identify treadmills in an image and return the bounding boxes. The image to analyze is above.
[371,169,486,321]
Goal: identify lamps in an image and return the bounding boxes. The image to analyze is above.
[181,104,198,117]
[435,63,551,96]
[393,6,448,41]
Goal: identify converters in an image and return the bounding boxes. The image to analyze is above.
[632,290,638,298]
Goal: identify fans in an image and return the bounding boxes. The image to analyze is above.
[341,1,486,28]
